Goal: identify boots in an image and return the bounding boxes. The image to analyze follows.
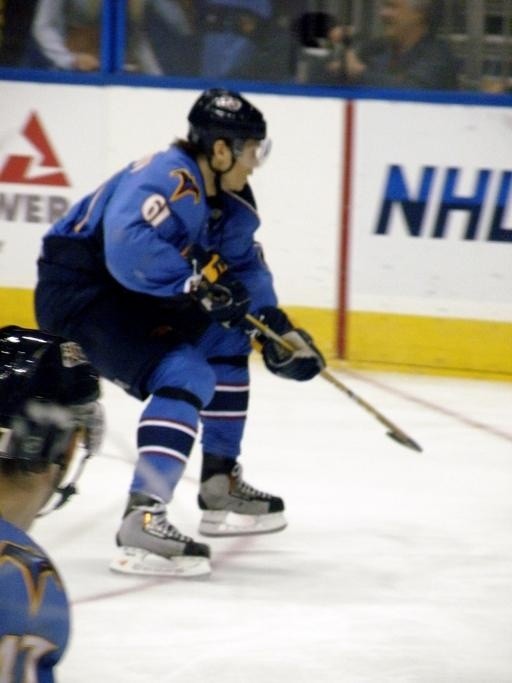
[115,492,210,559]
[197,452,284,515]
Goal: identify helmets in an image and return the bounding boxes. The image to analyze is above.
[0,325,101,466]
[186,87,266,157]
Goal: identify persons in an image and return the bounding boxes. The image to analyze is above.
[31,1,512,100]
[31,89,326,557]
[2,321,105,681]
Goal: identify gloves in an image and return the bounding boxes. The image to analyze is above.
[193,249,250,322]
[253,309,326,383]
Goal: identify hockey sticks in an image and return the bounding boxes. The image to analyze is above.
[245,314,422,453]
